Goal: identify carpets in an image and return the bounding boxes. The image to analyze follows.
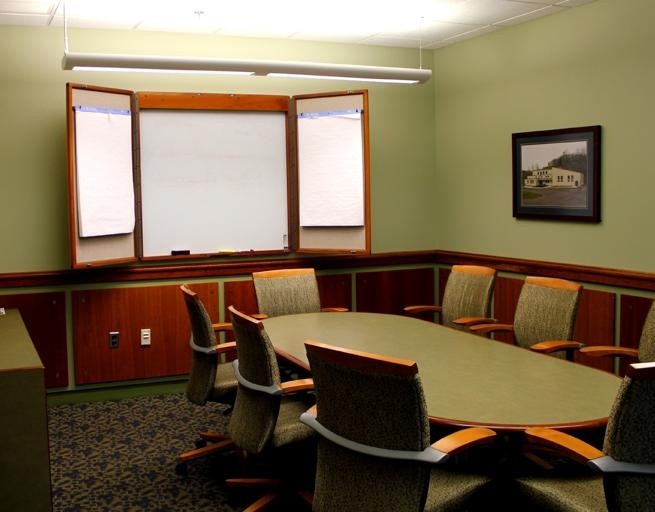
[53,391,311,512]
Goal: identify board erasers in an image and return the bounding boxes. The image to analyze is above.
[171,250,189,256]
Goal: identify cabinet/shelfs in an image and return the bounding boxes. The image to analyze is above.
[0,309,53,512]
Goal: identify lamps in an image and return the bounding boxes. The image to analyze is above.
[60,51,431,87]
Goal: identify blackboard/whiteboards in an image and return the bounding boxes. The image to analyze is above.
[139,108,289,258]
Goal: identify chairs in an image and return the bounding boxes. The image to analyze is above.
[513,361,655,512]
[579,300,655,361]
[175,284,239,474]
[470,275,586,362]
[403,264,497,340]
[252,268,350,382]
[298,340,498,511]
[224,306,315,511]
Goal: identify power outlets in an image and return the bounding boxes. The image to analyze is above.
[109,332,119,347]
[141,328,151,346]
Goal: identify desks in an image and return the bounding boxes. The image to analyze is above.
[258,311,623,473]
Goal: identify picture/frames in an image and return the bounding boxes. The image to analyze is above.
[511,125,601,224]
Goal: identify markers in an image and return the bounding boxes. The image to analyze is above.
[216,250,235,255]
[239,249,255,253]
